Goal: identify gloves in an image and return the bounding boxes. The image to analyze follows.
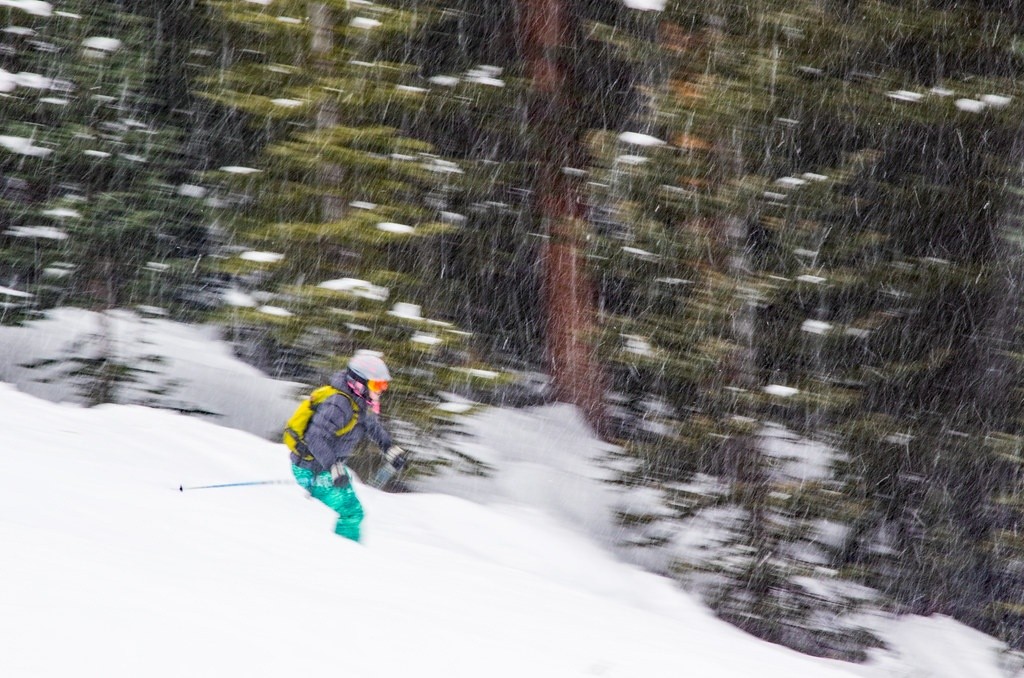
[334,475,348,487]
[392,457,409,471]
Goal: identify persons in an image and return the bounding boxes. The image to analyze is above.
[291,354,408,543]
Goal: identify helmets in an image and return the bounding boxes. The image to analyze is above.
[347,354,393,383]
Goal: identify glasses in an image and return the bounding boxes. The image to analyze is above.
[368,379,389,395]
